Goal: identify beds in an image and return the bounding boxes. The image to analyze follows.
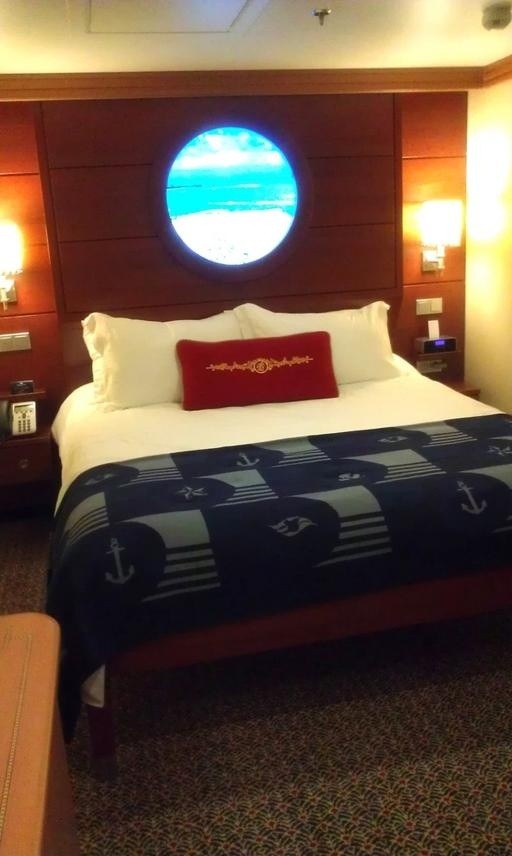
[44,298,512,782]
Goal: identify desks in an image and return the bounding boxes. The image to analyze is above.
[0,612,77,852]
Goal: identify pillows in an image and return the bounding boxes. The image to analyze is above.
[175,328,340,411]
[83,302,247,412]
[234,300,396,386]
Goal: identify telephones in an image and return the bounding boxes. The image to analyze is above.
[0,400,36,436]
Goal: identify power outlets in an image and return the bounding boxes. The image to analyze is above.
[0,332,32,353]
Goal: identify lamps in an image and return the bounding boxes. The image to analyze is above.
[480,0,512,31]
[420,197,463,273]
[0,220,26,303]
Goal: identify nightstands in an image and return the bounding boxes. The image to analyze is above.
[431,379,479,404]
[0,385,58,513]
